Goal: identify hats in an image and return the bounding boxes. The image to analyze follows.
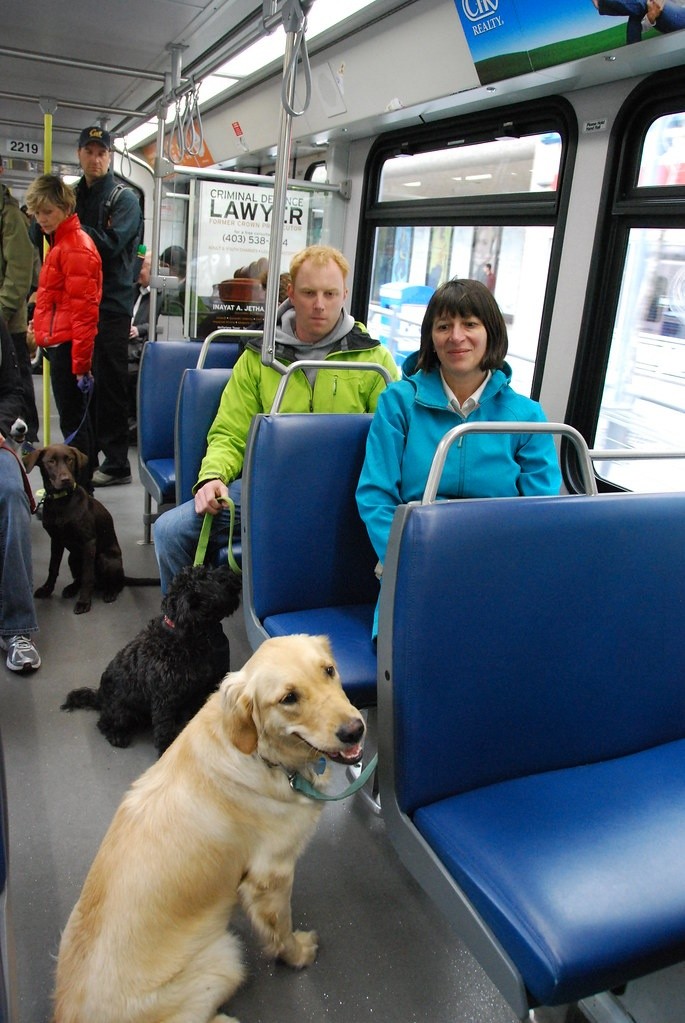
[79,126,110,150]
[161,246,187,266]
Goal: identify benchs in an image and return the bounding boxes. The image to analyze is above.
[135,329,684,1023]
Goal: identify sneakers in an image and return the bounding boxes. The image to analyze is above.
[0,634,41,671]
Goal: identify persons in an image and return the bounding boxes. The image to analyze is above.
[26,174,102,472]
[394,226,407,281]
[473,225,495,282]
[127,244,210,447]
[426,225,448,288]
[72,127,143,486]
[154,243,398,598]
[483,264,494,292]
[0,313,41,672]
[355,279,563,644]
[0,157,44,455]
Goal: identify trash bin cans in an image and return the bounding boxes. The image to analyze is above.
[379,282,433,367]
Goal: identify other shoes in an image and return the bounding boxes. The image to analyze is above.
[128,423,138,446]
[91,469,132,486]
[33,364,43,375]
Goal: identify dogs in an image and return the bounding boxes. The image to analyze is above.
[3,415,28,458]
[59,562,243,755]
[50,631,367,1023]
[22,443,161,614]
